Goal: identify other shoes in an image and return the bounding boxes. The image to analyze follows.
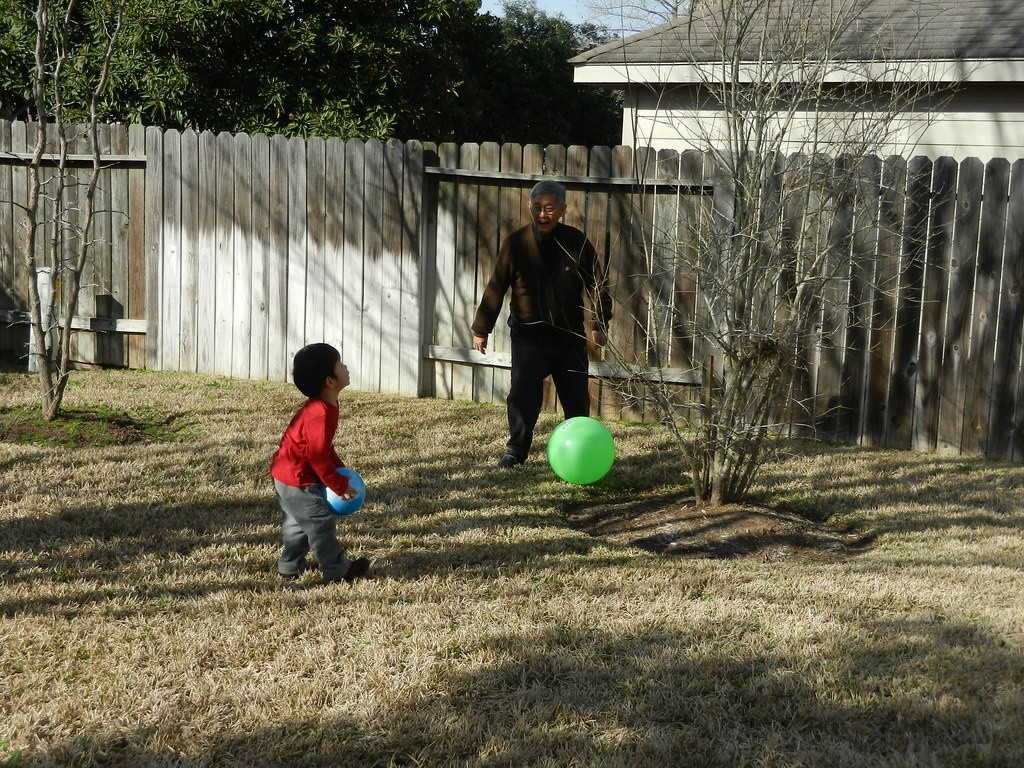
[498,452,525,468]
[336,557,370,582]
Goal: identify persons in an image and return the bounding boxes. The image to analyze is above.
[269,344,369,580]
[470,178,614,468]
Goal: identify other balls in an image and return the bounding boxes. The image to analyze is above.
[547,415,618,485]
[321,467,367,516]
